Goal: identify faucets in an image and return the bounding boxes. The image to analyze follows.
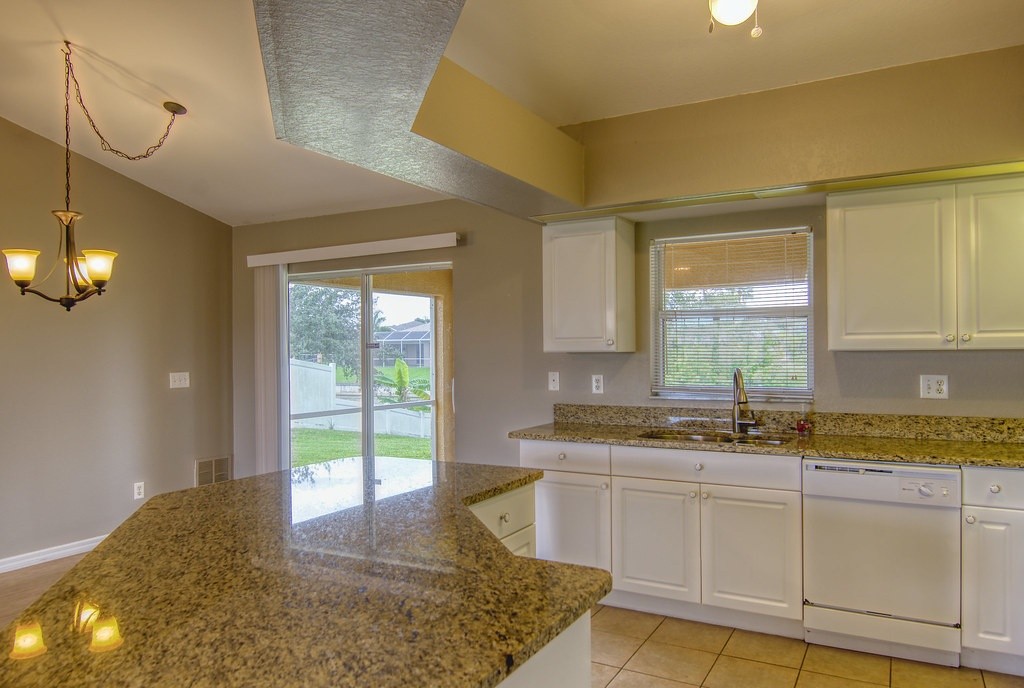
[731,368,757,435]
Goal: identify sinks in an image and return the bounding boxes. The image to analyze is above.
[633,429,795,447]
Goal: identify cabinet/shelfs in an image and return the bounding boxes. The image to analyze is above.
[498,607,593,688]
[826,176,1024,351]
[961,466,1023,676]
[468,483,537,558]
[541,216,636,352]
[520,439,803,640]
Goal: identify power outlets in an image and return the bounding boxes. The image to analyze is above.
[592,375,603,394]
[133,482,145,500]
[920,374,949,401]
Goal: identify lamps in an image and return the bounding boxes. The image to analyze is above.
[1,38,188,311]
[709,1,762,39]
